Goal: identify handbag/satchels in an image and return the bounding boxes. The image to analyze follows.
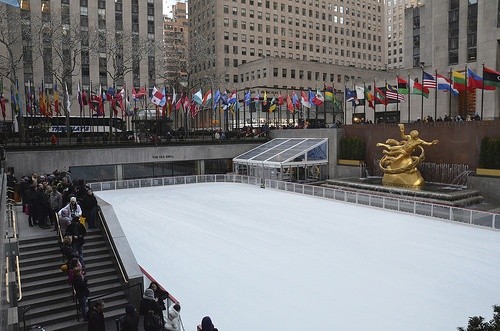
[25,204,31,214]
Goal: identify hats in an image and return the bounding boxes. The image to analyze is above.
[146,289,155,299]
[70,197,76,203]
[125,304,135,314]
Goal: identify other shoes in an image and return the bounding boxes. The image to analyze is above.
[29,224,33,227]
[33,222,38,224]
[52,229,57,231]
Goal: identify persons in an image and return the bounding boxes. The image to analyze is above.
[140,282,168,331]
[201,316,219,331]
[120,303,139,331]
[205,121,326,141]
[376,124,439,164]
[165,304,181,331]
[414,112,480,122]
[7,169,107,331]
[357,119,373,124]
[51,132,173,145]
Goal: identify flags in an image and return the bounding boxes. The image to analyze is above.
[467,68,483,89]
[483,67,500,87]
[374,87,389,105]
[437,73,459,96]
[397,78,409,94]
[409,79,430,99]
[0,85,386,117]
[423,72,437,88]
[453,71,466,89]
[386,83,404,102]
